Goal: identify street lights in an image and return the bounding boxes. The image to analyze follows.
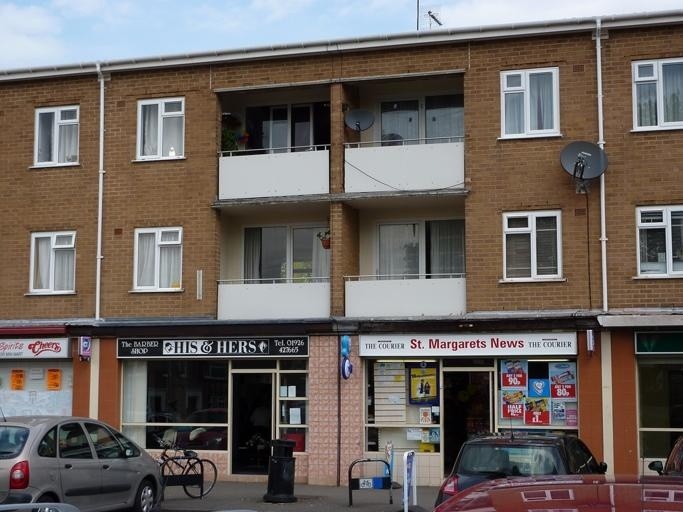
[329,315,341,488]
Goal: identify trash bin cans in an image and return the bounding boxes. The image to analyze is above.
[263,439,297,503]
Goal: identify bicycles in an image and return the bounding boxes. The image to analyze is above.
[152,433,218,501]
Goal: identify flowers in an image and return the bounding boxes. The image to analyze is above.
[316,230,331,239]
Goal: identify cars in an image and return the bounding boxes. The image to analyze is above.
[147,408,228,450]
[0,414,166,512]
[432,435,683,512]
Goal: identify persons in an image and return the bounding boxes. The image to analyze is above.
[416,379,430,398]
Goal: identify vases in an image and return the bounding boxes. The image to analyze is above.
[321,239,330,249]
